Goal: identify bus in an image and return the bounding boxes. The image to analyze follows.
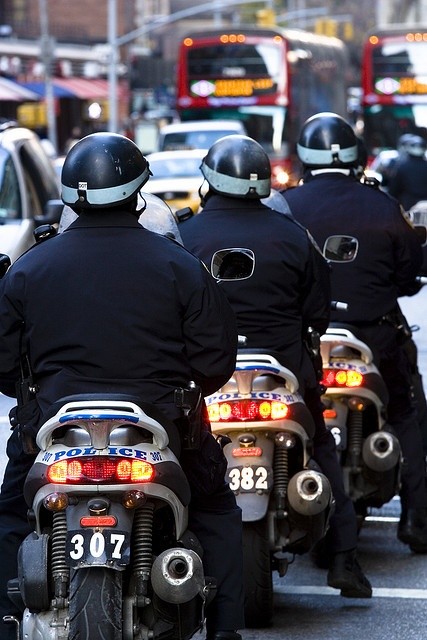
[175,29,351,169]
[360,29,426,126]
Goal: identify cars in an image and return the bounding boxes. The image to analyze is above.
[143,150,210,220]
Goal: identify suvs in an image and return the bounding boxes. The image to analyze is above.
[0,118,62,277]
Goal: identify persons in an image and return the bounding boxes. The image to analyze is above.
[175,135,372,599]
[382,133,427,213]
[266,111,427,557]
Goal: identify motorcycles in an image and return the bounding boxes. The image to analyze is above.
[175,189,359,629]
[320,201,427,568]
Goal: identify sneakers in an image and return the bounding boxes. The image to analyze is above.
[327,554,376,599]
[396,511,427,553]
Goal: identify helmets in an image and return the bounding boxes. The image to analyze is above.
[60,131,154,207]
[199,134,272,200]
[297,111,359,164]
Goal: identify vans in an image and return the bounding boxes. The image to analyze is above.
[161,120,247,154]
